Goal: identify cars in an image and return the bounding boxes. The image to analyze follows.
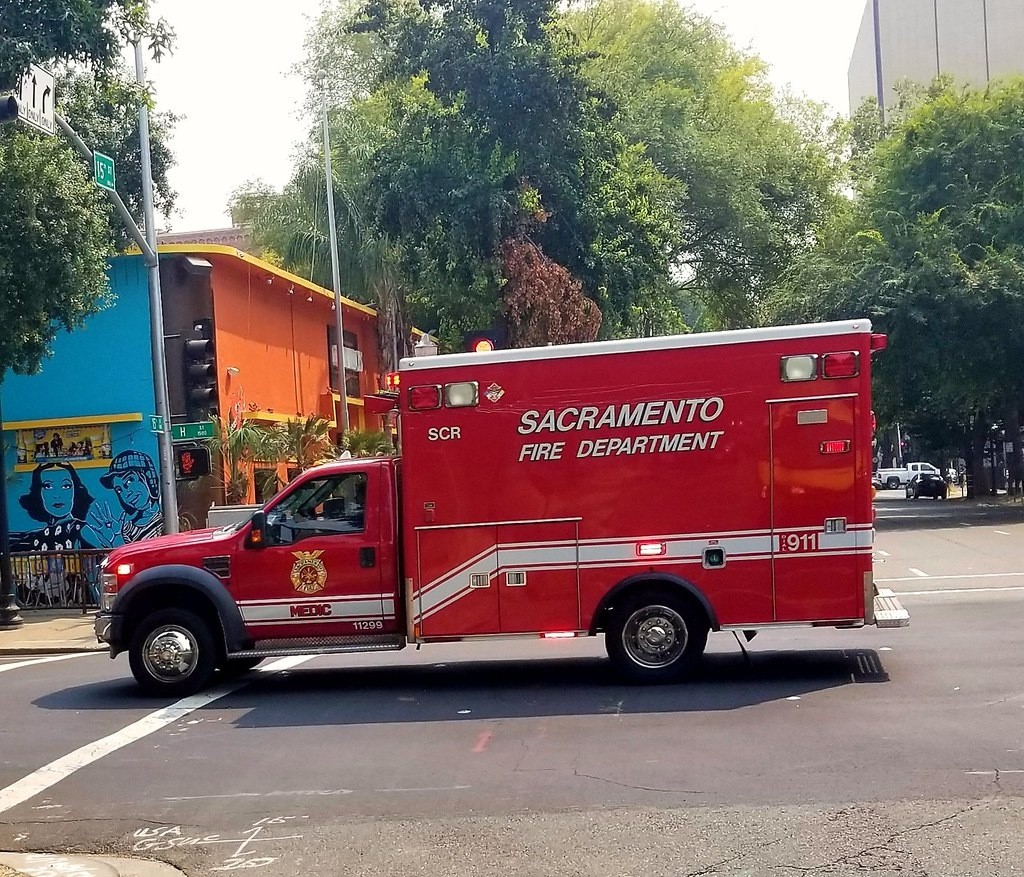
[905,473,947,500]
[872,478,882,490]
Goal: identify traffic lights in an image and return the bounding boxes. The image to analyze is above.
[1,94,20,122]
[900,440,907,446]
[465,330,503,353]
[174,447,211,480]
[181,338,219,419]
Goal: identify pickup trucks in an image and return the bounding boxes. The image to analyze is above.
[876,462,956,489]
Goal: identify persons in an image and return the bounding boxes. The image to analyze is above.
[960,465,966,485]
[351,482,367,527]
[36,433,92,457]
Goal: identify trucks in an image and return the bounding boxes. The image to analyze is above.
[94,318,910,684]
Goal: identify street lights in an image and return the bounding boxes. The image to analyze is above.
[318,68,350,436]
